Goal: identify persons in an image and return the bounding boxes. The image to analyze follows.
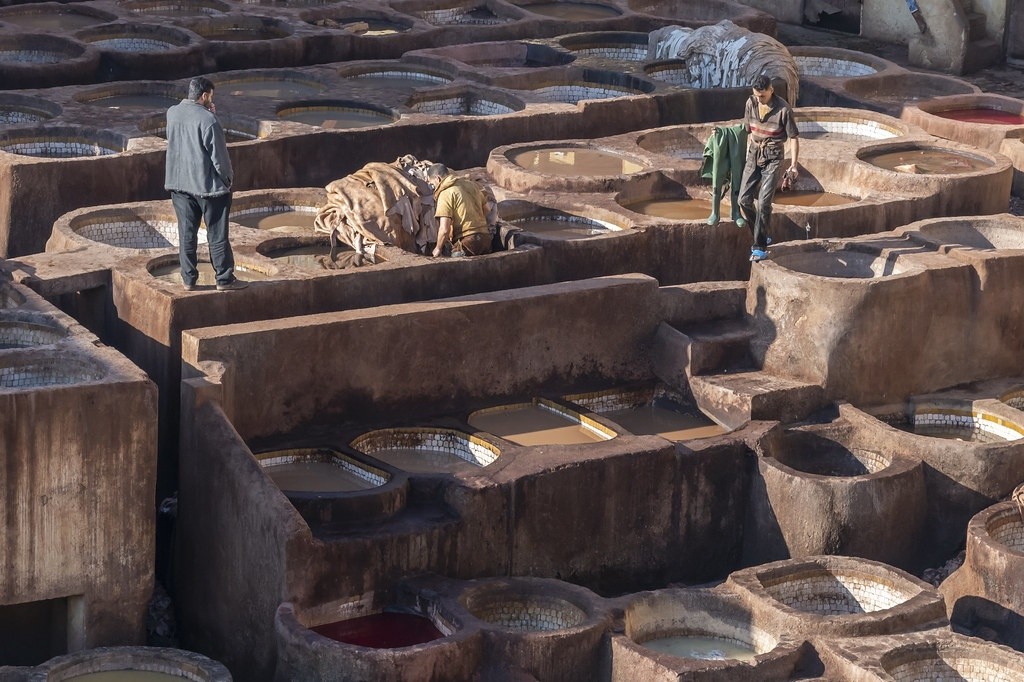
[427,163,493,259]
[711,75,799,262]
[163,77,248,291]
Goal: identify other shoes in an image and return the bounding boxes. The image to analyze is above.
[219,279,248,290]
[182,278,195,290]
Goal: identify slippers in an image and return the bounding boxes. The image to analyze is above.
[752,238,771,252]
[751,249,767,261]
